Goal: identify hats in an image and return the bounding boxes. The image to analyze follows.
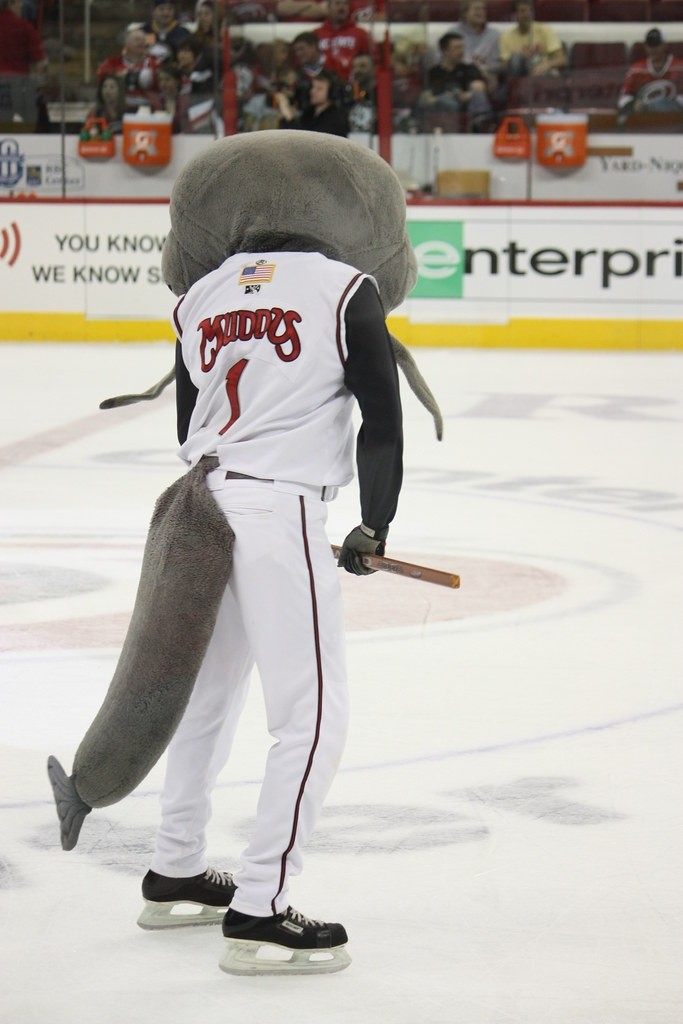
[645,29,661,47]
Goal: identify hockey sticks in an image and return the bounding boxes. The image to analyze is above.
[330,539,461,593]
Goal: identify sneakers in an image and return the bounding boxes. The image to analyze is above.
[137,867,238,928]
[220,905,352,972]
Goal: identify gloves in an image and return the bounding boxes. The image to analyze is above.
[337,521,389,576]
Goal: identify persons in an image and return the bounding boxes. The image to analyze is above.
[87,0,682,139]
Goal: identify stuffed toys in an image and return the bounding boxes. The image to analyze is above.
[48,127,442,978]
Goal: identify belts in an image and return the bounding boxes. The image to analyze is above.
[225,471,335,503]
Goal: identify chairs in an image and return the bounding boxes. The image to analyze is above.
[392,41,683,134]
